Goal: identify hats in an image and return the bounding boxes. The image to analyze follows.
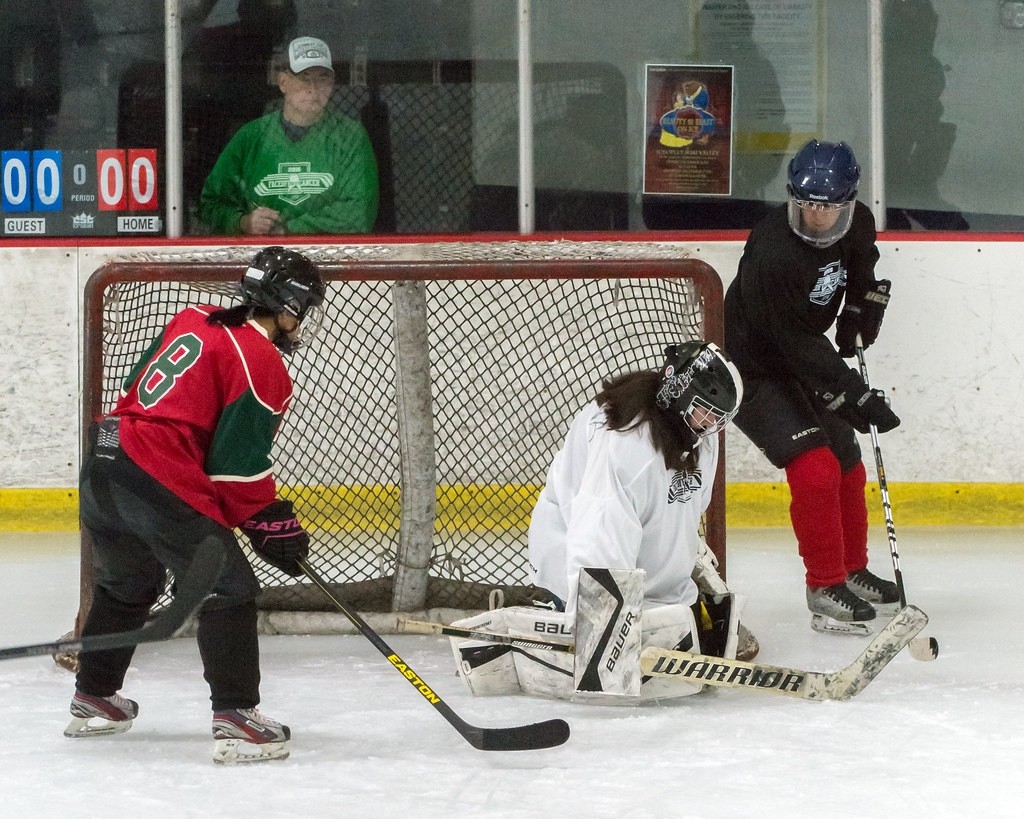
[282,37,335,74]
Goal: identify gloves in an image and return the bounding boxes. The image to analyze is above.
[813,368,901,434]
[238,500,310,576]
[837,279,891,358]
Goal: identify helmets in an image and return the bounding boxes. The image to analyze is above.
[655,338,744,443]
[240,244,328,354]
[784,139,861,207]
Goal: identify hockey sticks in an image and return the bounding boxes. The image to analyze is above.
[0,533,226,662]
[855,332,940,661]
[295,555,570,751]
[392,603,929,703]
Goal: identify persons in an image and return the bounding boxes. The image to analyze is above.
[449,340,744,704]
[880,0,970,230]
[200,35,380,235]
[642,0,790,231]
[129,1,299,236]
[62,245,328,766]
[722,139,901,637]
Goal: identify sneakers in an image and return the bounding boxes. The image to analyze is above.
[842,567,903,617]
[807,583,877,637]
[211,706,290,764]
[64,673,140,738]
